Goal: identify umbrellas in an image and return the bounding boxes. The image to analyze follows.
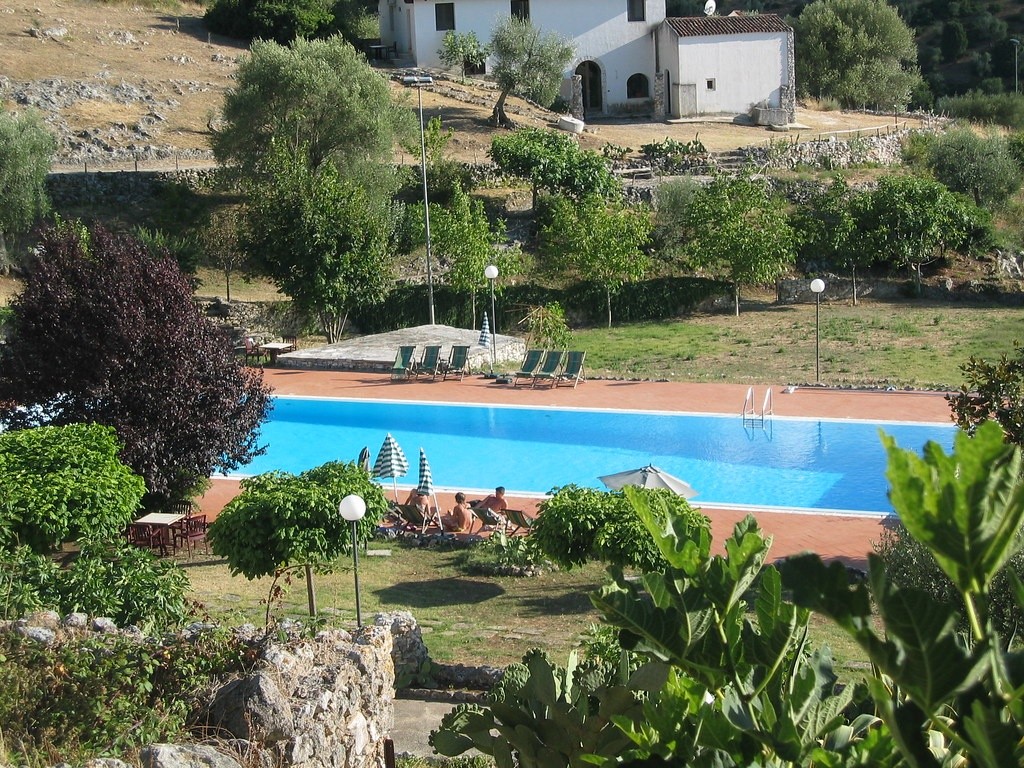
[372,432,409,505]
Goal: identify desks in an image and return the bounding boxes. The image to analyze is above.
[135,512,185,554]
[259,342,293,364]
[370,44,387,59]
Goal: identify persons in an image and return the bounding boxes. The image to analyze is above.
[399,489,420,529]
[442,492,472,532]
[406,489,430,531]
[474,486,508,522]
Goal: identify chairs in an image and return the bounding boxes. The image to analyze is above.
[393,504,442,534]
[512,349,546,388]
[388,345,418,382]
[443,345,473,383]
[245,339,268,365]
[278,336,297,354]
[501,508,536,538]
[167,500,191,548]
[387,41,399,59]
[416,345,444,381]
[125,523,165,559]
[532,350,566,390]
[173,515,210,561]
[467,507,514,539]
[556,351,588,389]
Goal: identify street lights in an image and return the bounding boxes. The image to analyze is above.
[403,76,439,323]
[1010,38,1020,92]
[485,265,500,364]
[339,495,366,629]
[809,278,826,381]
